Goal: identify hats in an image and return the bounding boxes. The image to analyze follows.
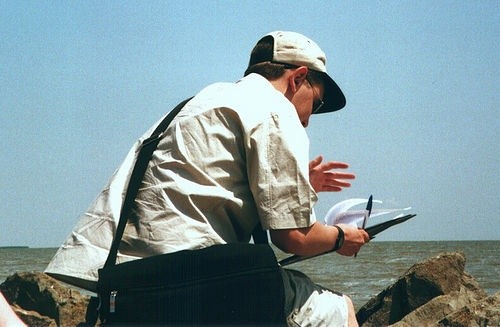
[244,31,346,113]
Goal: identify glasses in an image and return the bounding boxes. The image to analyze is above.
[305,77,324,115]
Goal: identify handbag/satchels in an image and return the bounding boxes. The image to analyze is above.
[83,97,289,326]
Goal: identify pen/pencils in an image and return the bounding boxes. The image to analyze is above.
[353,194,375,261]
